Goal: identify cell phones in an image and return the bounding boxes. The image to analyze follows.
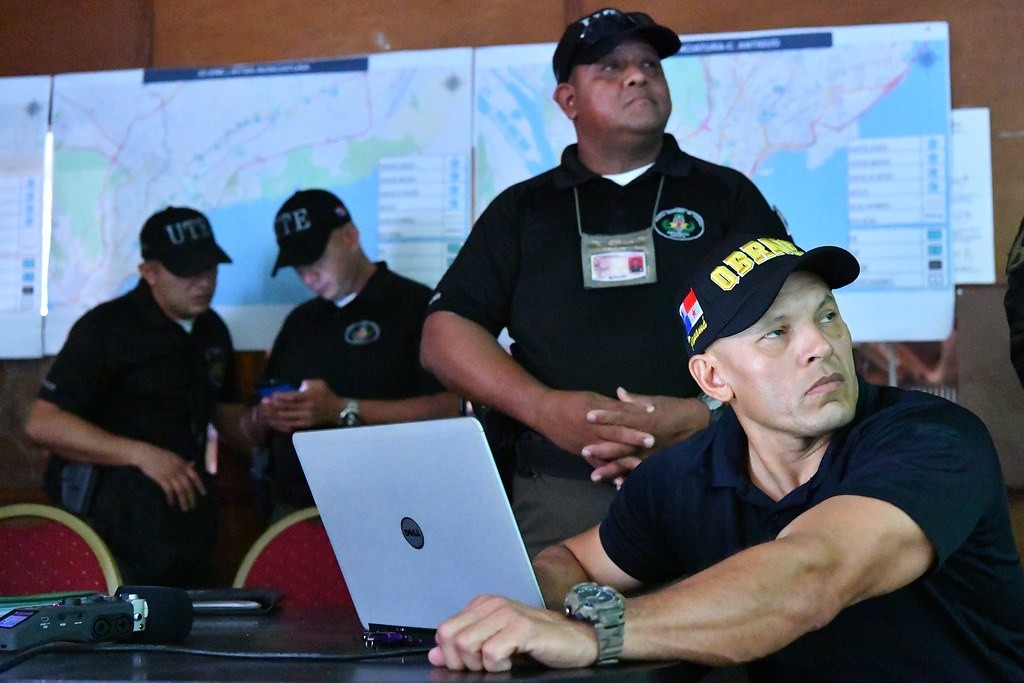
[254,381,295,399]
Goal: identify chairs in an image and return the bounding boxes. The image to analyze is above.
[231,504,358,611]
[0,503,123,599]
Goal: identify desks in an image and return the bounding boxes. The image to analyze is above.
[0,591,682,683]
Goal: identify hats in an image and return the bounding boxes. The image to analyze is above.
[674,231,860,360]
[270,188,352,278]
[552,7,682,84]
[139,206,232,277]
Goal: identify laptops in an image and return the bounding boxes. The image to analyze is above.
[293,417,546,646]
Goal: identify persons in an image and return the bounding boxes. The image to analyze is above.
[427,221,1024,683]
[18,203,253,622]
[1003,223,1023,380]
[419,8,808,579]
[238,182,434,559]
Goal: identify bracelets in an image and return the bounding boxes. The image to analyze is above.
[339,394,362,430]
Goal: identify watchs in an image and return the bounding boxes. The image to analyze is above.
[561,580,630,670]
[696,391,727,432]
